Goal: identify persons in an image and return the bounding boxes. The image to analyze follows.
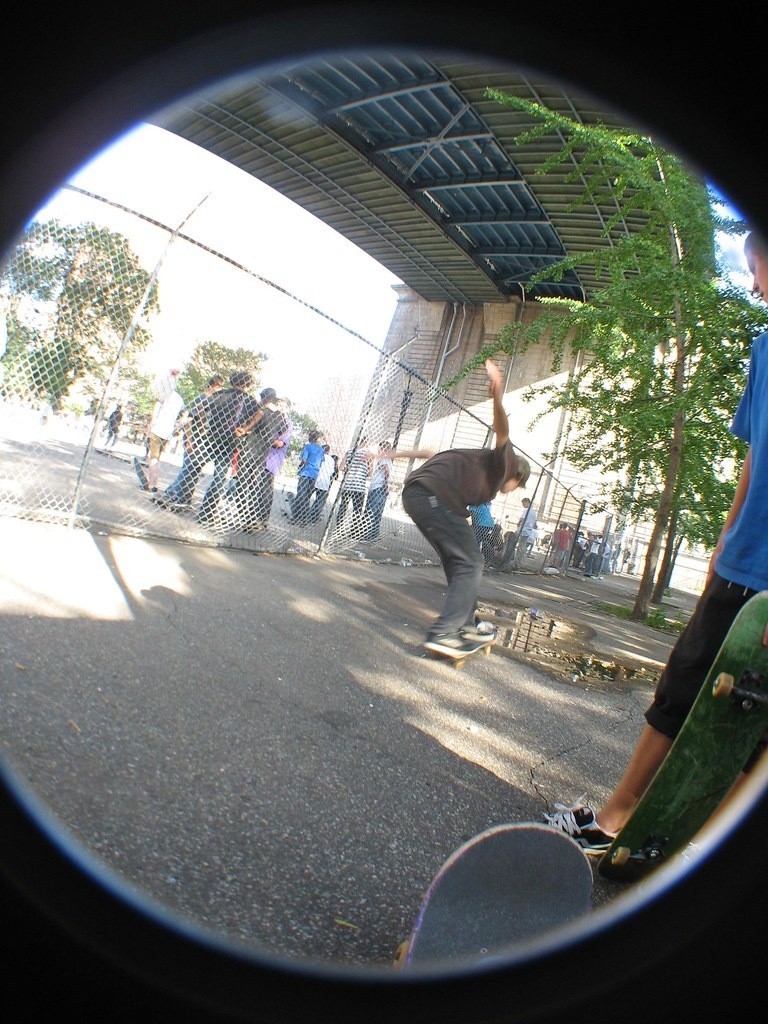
[88,371,394,546]
[541,231,768,856]
[467,497,634,580]
[386,359,531,654]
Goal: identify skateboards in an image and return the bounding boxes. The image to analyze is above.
[134,457,149,490]
[393,822,594,968]
[597,586,766,880]
[421,624,500,666]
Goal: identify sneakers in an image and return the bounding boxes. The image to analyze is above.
[462,620,495,642]
[425,631,478,656]
[541,791,620,854]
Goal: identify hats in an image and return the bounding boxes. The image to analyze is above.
[514,454,532,490]
